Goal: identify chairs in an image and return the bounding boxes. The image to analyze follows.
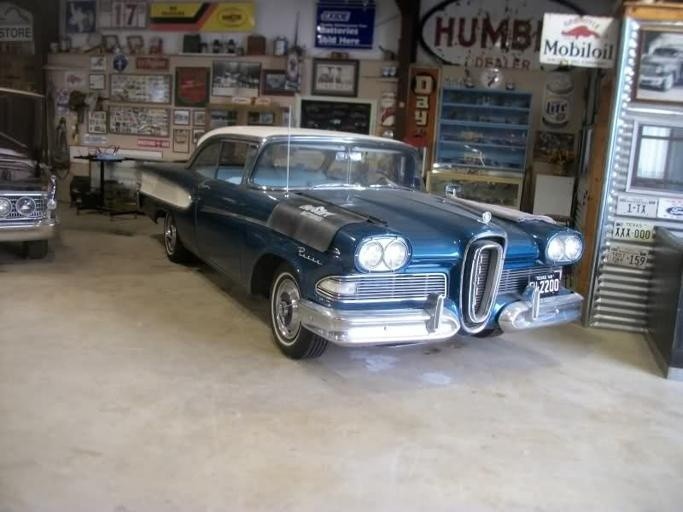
[219,158,376,187]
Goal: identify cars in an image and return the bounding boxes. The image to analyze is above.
[134,123,583,359]
[2,135,57,259]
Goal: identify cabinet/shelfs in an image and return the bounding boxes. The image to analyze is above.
[435,87,534,172]
[425,169,523,211]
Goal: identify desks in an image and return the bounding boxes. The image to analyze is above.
[130,157,189,209]
[73,151,135,217]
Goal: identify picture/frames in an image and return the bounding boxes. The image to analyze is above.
[88,34,296,154]
[311,58,359,97]
